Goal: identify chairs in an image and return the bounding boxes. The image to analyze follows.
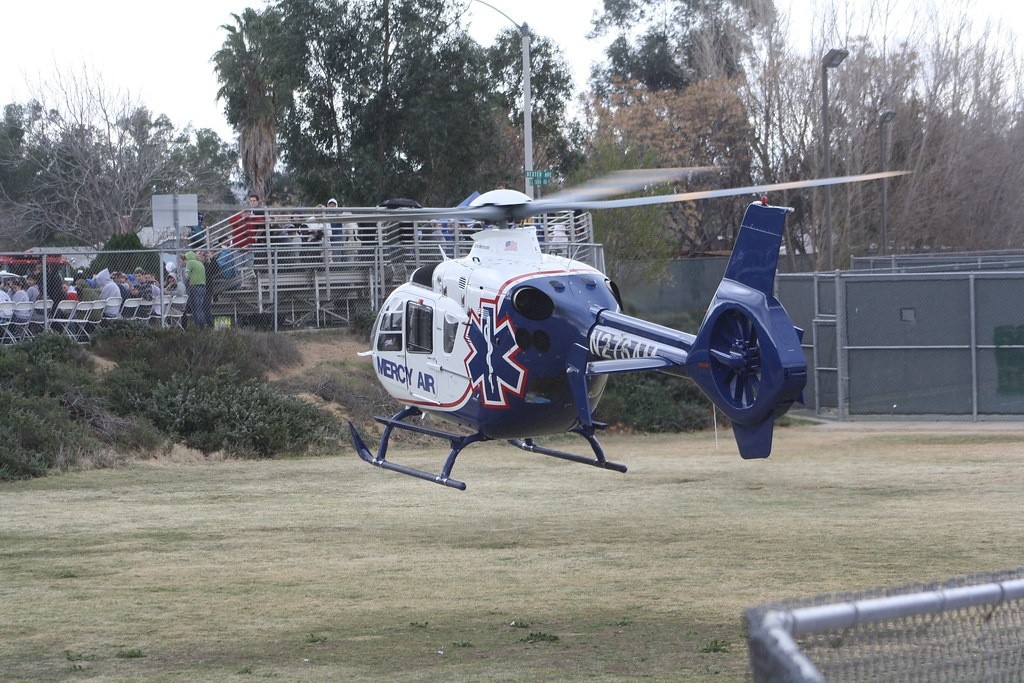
[0,295,189,346]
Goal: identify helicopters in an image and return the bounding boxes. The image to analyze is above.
[117,164,917,492]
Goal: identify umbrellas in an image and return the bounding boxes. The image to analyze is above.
[379,198,422,208]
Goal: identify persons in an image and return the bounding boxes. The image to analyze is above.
[96,268,122,328]
[192,195,376,279]
[391,182,584,260]
[185,251,205,328]
[0,251,218,341]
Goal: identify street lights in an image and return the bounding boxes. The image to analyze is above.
[879,110,897,257]
[820,47,852,272]
